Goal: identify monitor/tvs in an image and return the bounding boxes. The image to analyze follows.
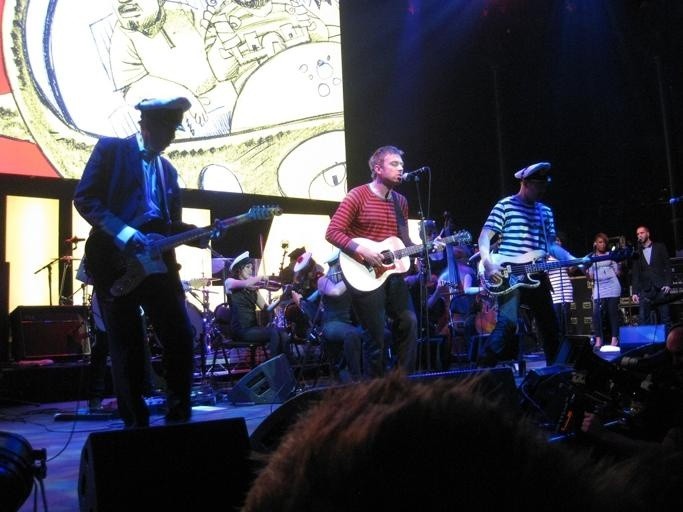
[2,0,351,216]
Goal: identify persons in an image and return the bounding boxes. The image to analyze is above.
[75,98,229,428]
[547,236,575,348]
[283,247,370,383]
[587,235,623,353]
[632,225,672,340]
[478,161,589,366]
[244,376,566,511]
[326,146,447,375]
[225,252,291,359]
[581,445,682,512]
[404,220,501,345]
[579,323,683,453]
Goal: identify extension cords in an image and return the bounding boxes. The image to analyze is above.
[63,238,88,244]
[54,413,113,420]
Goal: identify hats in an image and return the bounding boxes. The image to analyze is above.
[515,163,554,183]
[293,251,315,275]
[229,251,255,272]
[288,246,305,259]
[134,97,192,111]
[469,250,494,266]
[327,252,340,267]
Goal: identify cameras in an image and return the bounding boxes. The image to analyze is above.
[518,336,678,443]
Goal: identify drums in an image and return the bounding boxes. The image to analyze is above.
[183,304,207,354]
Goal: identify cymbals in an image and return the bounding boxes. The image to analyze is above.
[56,255,82,261]
[66,237,84,243]
[191,276,221,285]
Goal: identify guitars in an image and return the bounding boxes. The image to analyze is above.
[87,205,281,301]
[476,245,639,297]
[340,229,471,292]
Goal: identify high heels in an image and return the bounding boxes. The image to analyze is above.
[594,344,601,348]
[611,340,618,346]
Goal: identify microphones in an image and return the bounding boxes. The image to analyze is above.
[585,242,598,255]
[261,279,280,286]
[403,166,428,183]
[634,238,643,246]
[446,280,460,289]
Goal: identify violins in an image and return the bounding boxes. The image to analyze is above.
[420,272,458,290]
[244,273,282,292]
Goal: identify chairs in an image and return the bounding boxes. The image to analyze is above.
[209,302,270,388]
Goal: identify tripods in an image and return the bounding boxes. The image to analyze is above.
[413,176,432,377]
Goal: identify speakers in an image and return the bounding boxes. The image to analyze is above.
[78,417,249,512]
[417,335,448,372]
[512,333,538,357]
